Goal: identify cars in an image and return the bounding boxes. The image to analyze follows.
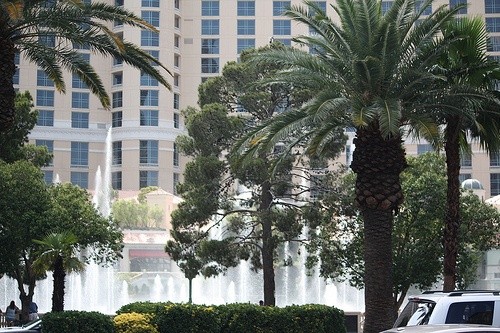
[378,325,500,333]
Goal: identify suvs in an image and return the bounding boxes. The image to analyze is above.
[392,290,500,328]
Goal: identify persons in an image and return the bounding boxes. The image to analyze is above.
[4,298,39,326]
